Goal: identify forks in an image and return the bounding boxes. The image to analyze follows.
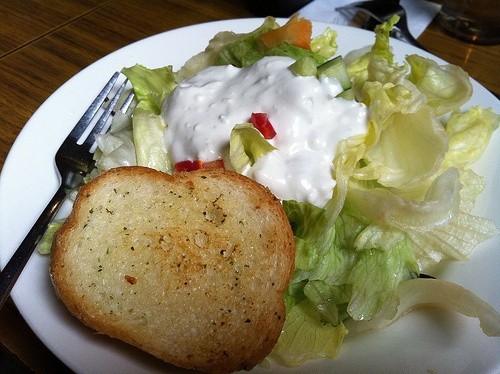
[335,0,432,53]
[0,72,141,308]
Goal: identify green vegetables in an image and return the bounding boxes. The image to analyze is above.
[35,218,70,257]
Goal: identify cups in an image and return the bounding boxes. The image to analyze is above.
[439,0,500,45]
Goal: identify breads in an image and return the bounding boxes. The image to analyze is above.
[50,165,297,372]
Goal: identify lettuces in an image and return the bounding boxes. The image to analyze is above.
[85,18,500,366]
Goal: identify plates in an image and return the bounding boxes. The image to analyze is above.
[0,16,500,373]
